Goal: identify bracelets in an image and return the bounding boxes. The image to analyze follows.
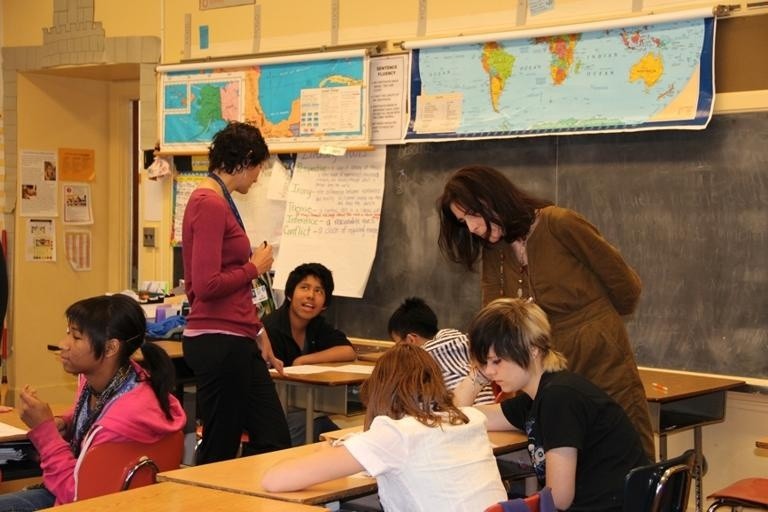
[470,364,491,391]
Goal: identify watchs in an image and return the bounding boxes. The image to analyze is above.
[182,122,292,466]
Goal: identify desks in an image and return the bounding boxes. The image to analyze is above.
[35,483,333,512]
[636,367,744,512]
[157,439,377,506]
[320,420,530,460]
[266,361,382,444]
[47,335,198,411]
[0,402,73,492]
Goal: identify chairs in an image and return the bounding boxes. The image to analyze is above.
[625,452,697,512]
[487,382,514,406]
[72,426,183,503]
[707,438,768,512]
[485,487,556,512]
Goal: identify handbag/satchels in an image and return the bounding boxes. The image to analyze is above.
[145,315,187,340]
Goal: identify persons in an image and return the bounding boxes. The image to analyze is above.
[451,297,652,511]
[433,161,642,511]
[23,184,35,199]
[260,345,510,512]
[256,259,357,368]
[44,161,56,180]
[0,293,185,511]
[388,297,499,406]
